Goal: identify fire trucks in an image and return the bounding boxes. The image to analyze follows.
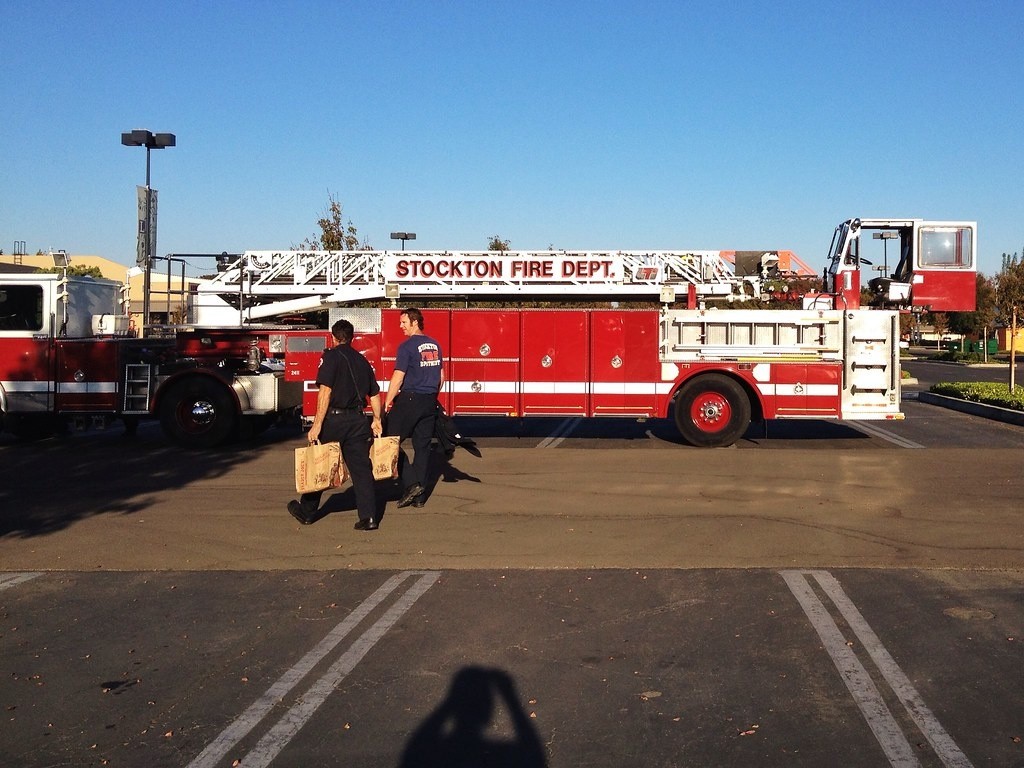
[0,218,977,453]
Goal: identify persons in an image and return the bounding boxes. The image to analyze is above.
[287,320,383,530]
[381,309,445,508]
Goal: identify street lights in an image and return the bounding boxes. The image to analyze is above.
[121,129,177,339]
[390,232,417,251]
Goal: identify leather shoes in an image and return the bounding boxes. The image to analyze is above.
[287,500,311,524]
[410,498,424,508]
[353,516,378,531]
[396,483,424,508]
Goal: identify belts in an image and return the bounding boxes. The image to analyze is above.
[328,406,360,415]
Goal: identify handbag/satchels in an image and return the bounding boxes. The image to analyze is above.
[294,438,350,494]
[368,428,400,481]
